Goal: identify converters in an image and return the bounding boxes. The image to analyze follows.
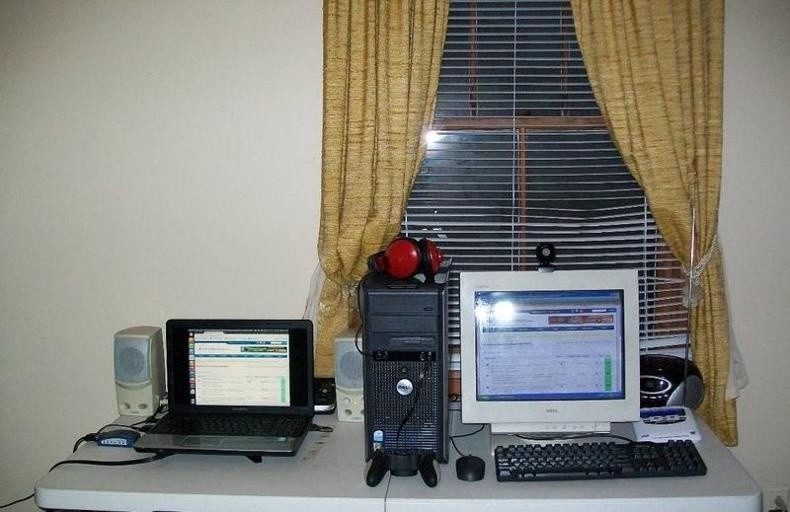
[98,430,140,447]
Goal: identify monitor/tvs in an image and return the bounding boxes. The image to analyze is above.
[460,268,640,455]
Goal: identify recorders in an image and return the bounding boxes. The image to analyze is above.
[639,354,704,411]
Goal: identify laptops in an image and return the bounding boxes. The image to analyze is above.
[133,319,315,456]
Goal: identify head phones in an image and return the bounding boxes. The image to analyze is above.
[368,238,443,279]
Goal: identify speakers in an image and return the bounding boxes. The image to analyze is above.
[334,328,364,423]
[115,325,165,417]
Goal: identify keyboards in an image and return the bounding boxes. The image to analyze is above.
[495,439,709,483]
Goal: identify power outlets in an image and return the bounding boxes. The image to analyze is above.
[769,486,788,510]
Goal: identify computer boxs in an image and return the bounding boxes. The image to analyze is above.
[360,267,449,463]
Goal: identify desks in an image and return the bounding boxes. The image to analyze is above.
[34,399,762,511]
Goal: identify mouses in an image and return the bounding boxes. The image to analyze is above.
[456,456,485,481]
